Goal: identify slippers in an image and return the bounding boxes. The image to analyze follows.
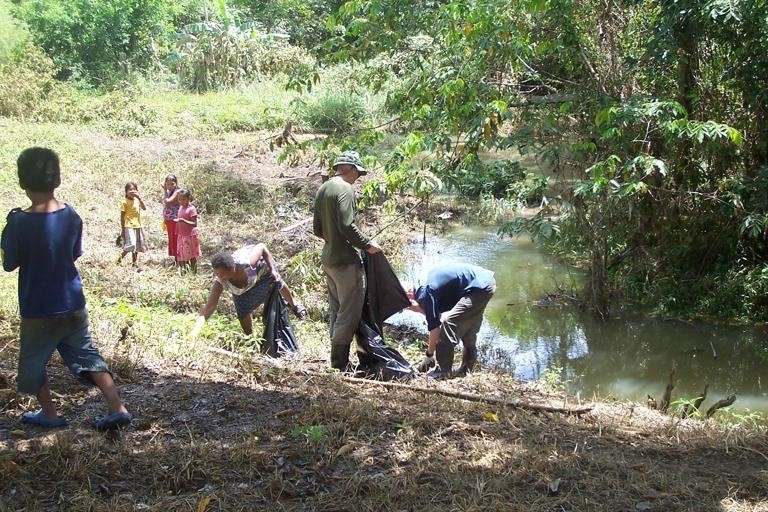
[91,412,131,428]
[287,302,307,320]
[22,408,65,426]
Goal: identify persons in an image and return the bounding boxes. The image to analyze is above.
[313,150,383,375]
[3,146,132,431]
[188,242,306,347]
[400,260,496,381]
[174,190,202,275]
[161,174,184,266]
[119,180,147,266]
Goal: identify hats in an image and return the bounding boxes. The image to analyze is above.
[333,151,367,176]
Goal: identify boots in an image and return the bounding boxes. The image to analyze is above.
[427,341,453,381]
[330,341,363,378]
[455,348,477,377]
